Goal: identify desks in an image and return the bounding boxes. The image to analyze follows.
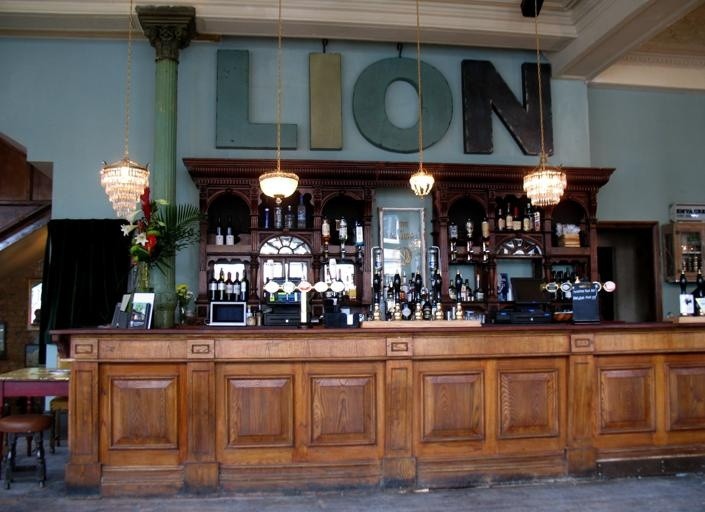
[0,362,69,460]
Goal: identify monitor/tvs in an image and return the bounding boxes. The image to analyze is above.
[509,277,552,313]
[266,276,303,313]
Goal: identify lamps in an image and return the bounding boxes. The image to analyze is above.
[402,1,441,200]
[260,0,300,206]
[98,1,154,222]
[519,2,568,211]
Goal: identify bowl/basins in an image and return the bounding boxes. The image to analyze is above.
[552,312,574,321]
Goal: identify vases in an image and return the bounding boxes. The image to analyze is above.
[178,302,189,325]
[133,260,149,294]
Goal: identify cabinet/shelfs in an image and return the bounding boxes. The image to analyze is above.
[181,158,381,310]
[661,213,705,289]
[424,158,616,314]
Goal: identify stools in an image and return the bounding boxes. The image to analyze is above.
[47,390,71,456]
[1,411,53,491]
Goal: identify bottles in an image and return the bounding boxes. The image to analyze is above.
[246,304,262,326]
[262,195,308,229]
[369,266,483,320]
[325,266,346,299]
[448,217,490,246]
[215,216,235,245]
[552,270,587,301]
[321,214,364,245]
[580,220,587,247]
[683,256,699,272]
[496,200,542,232]
[207,270,249,302]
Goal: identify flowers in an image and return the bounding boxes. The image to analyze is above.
[121,180,204,270]
[173,283,193,301]
